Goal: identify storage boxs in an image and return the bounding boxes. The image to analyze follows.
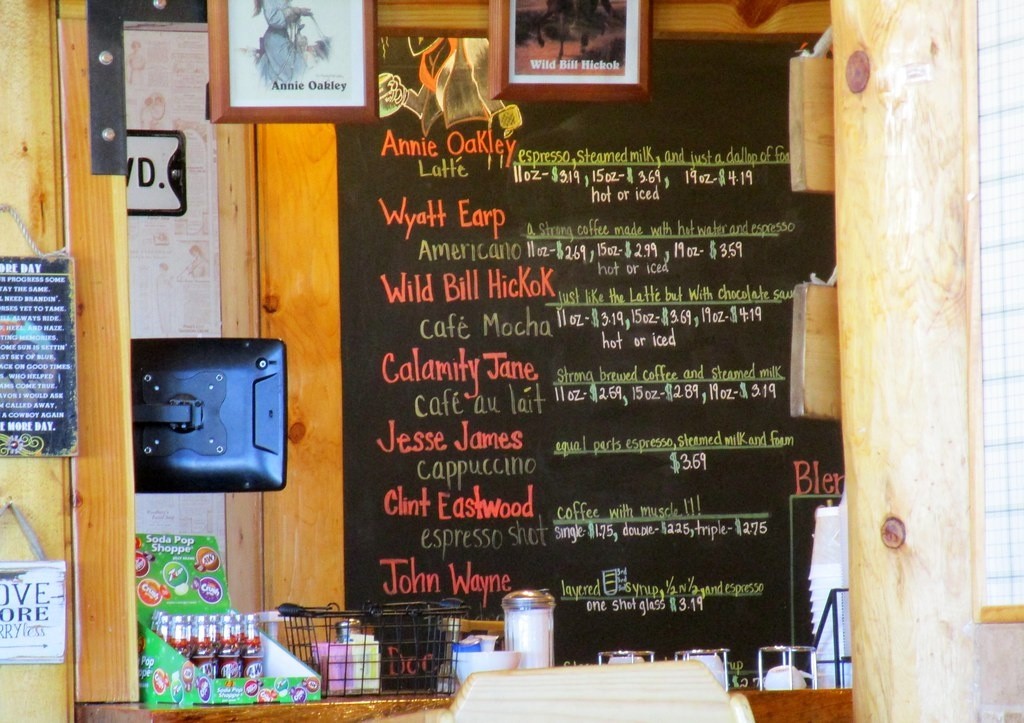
[138,608,323,705]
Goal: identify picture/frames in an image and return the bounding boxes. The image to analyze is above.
[207,0,381,125]
[488,0,652,103]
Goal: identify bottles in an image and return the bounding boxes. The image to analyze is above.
[502,587,555,670]
[150,610,265,680]
[336,618,361,643]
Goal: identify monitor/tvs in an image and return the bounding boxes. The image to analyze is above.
[131,337,288,492]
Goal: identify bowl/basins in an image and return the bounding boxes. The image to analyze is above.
[452,652,522,687]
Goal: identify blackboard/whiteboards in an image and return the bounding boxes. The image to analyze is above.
[336,34,845,691]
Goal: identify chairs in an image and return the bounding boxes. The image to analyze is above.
[360,659,756,723]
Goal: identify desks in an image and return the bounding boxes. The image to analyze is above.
[74,688,852,723]
